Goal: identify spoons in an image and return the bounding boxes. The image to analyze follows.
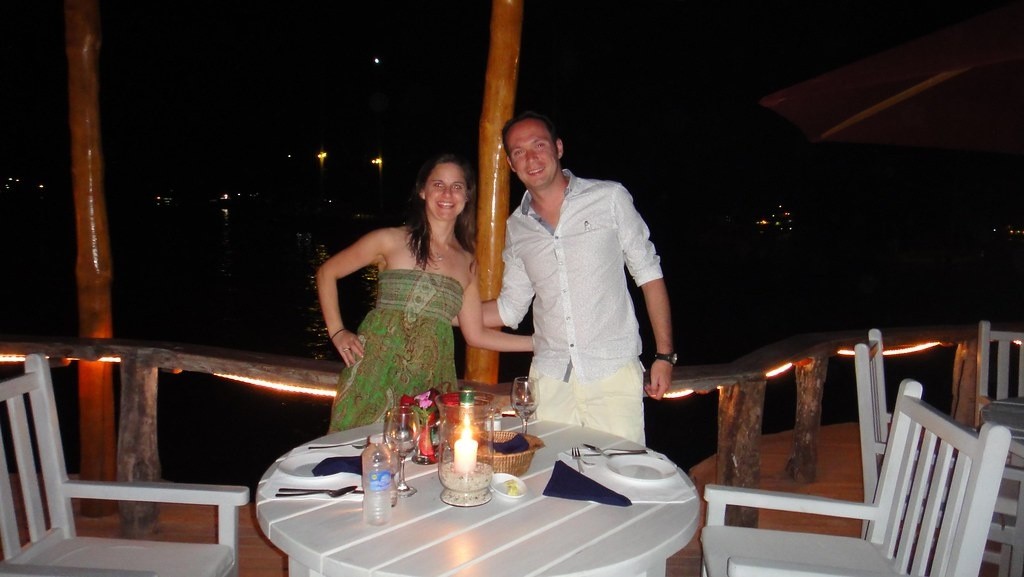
[562,449,596,465]
[583,443,646,453]
[275,485,358,498]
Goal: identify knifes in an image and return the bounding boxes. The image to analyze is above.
[279,488,365,494]
[584,452,648,456]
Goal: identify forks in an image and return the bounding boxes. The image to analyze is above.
[571,446,584,473]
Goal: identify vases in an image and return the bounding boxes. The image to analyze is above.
[412,409,439,465]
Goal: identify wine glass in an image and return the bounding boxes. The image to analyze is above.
[510,376,540,434]
[383,406,419,498]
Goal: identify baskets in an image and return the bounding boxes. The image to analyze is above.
[476,431,544,477]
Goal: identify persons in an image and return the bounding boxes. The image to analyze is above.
[453,112,674,445]
[316,156,532,434]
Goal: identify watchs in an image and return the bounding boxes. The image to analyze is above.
[655,353,677,364]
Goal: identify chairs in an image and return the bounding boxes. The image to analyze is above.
[0,352,250,577]
[701,378,1010,577]
[852,320,1024,577]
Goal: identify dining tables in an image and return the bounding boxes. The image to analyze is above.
[255,410,704,577]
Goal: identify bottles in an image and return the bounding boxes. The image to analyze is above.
[361,434,398,526]
[450,384,482,462]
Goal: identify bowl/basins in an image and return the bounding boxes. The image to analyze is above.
[490,473,528,501]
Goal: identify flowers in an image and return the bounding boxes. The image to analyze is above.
[399,388,441,425]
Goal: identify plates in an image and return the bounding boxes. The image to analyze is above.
[276,453,341,480]
[606,454,678,482]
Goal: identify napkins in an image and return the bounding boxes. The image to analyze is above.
[542,460,634,507]
[311,452,362,476]
[492,430,529,454]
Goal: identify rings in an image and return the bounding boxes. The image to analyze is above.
[344,349,349,351]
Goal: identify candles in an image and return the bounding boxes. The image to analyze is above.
[453,416,478,470]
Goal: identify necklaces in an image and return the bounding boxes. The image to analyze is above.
[439,257,442,260]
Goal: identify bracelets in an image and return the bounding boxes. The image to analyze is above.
[330,328,346,338]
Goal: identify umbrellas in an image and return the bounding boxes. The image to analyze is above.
[757,0,1024,152]
